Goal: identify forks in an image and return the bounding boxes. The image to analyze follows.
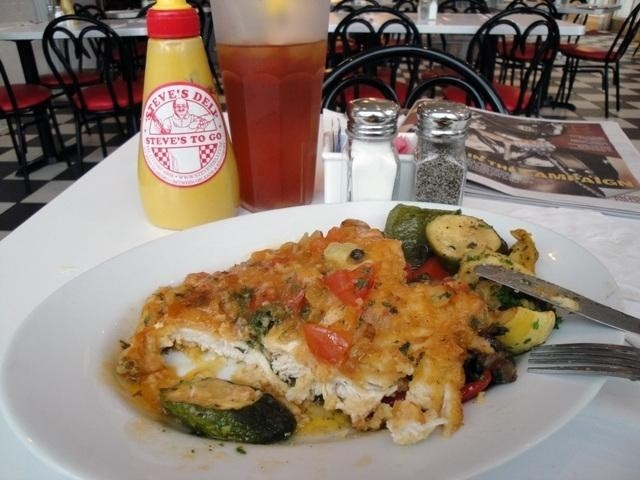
[524,342,640,379]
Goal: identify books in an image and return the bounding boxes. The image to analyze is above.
[390,96,640,218]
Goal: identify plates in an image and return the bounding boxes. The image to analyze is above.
[0,201,627,480]
[106,10,139,19]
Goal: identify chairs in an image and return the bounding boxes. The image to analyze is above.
[2,1,222,190]
[321,2,638,119]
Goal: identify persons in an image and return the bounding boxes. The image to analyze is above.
[152,97,211,136]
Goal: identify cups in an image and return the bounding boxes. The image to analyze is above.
[208,0,331,214]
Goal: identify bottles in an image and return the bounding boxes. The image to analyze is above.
[414,101,467,204]
[136,0,241,231]
[47,5,66,28]
[418,1,437,22]
[60,0,76,24]
[343,98,401,200]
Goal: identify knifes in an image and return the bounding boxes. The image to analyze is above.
[475,265,640,336]
